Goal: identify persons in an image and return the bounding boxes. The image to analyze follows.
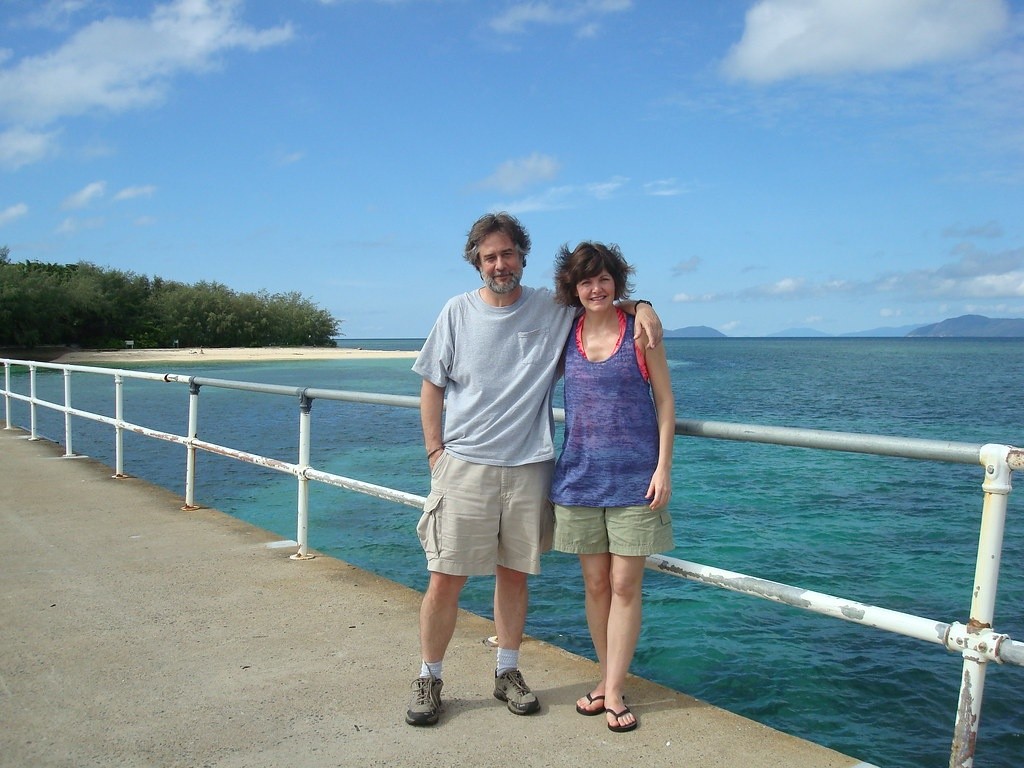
[405,213,664,728]
[552,239,675,733]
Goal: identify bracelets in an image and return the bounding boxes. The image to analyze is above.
[428,446,443,459]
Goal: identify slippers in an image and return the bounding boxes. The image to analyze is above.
[576,692,625,716]
[605,705,637,732]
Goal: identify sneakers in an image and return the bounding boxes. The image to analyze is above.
[493,667,540,715]
[405,661,444,726]
